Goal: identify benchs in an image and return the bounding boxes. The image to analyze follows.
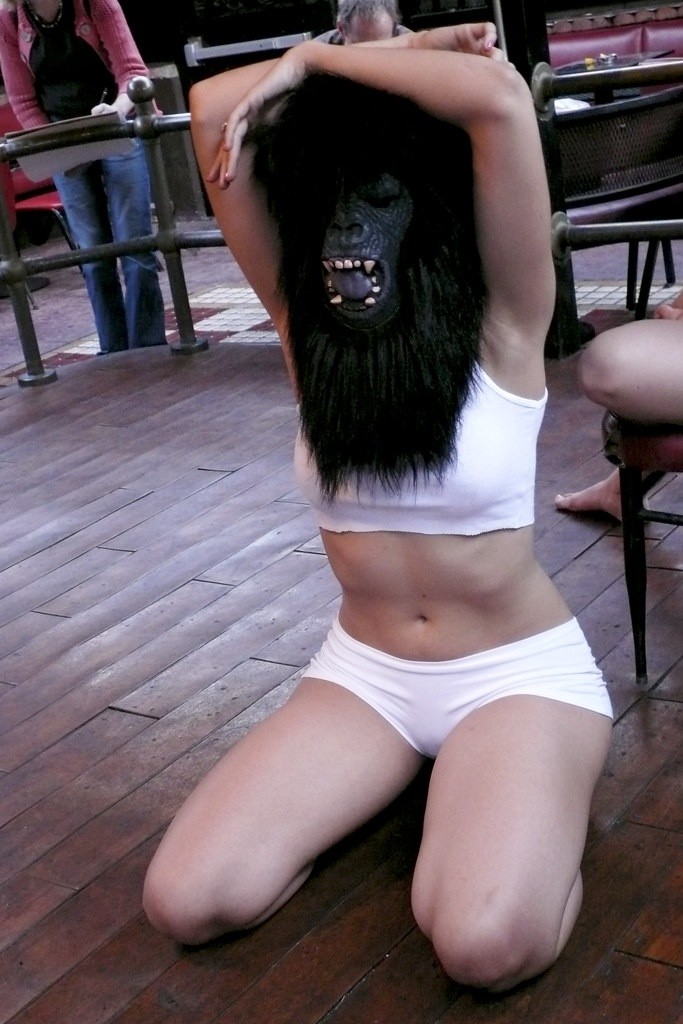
[555,86,683,321]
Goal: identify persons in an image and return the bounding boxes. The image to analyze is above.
[312,1,413,46]
[140,20,615,994]
[554,291,681,522]
[2,1,168,352]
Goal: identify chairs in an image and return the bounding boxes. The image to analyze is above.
[0,95,77,273]
[600,409,683,686]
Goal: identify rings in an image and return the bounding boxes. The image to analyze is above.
[220,122,227,133]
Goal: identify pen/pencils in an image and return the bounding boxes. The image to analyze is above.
[100,87,108,103]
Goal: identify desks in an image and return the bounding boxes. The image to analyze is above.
[552,49,675,105]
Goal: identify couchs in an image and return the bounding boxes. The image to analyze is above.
[547,19,683,97]
